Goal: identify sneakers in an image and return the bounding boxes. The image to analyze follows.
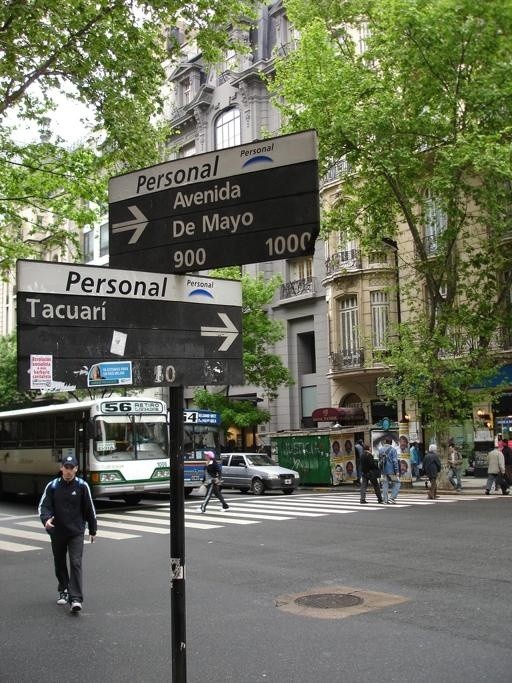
[70,600,81,613]
[503,490,510,494]
[485,488,490,494]
[57,591,69,604]
[427,492,435,498]
[360,498,397,505]
[223,504,228,509]
[201,504,206,512]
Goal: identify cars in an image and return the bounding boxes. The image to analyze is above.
[220,452,300,495]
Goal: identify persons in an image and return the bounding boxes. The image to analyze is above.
[484,437,511,496]
[199,451,229,512]
[330,439,356,485]
[398,460,411,480]
[410,442,421,481]
[379,437,401,504]
[399,435,409,454]
[423,444,441,498]
[361,444,382,504]
[448,443,464,492]
[37,456,97,612]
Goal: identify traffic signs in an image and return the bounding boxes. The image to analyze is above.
[107,127,321,274]
[15,258,246,392]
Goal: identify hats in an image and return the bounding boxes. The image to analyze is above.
[63,456,79,467]
[203,451,214,458]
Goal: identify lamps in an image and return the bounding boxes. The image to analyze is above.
[477,408,490,417]
[483,421,493,428]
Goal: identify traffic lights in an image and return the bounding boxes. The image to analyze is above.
[478,410,494,430]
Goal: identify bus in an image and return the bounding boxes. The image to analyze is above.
[0,397,170,505]
[167,405,223,497]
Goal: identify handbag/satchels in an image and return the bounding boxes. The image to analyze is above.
[377,452,387,470]
[369,469,381,478]
